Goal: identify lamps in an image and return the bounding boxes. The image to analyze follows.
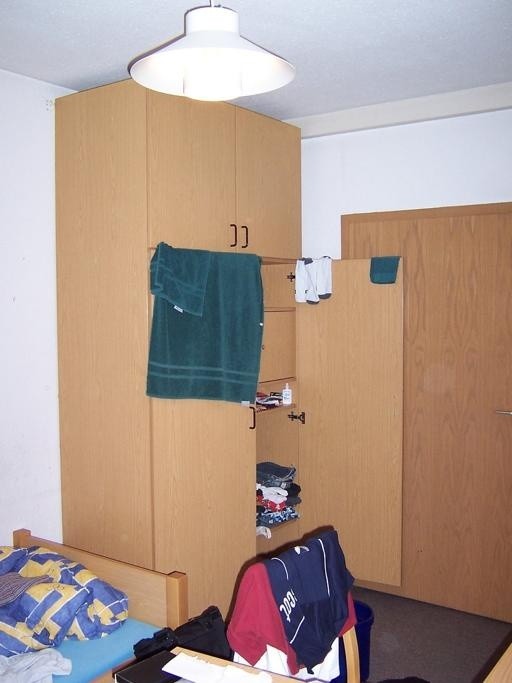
[128,0,298,102]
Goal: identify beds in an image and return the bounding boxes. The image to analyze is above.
[0,528,189,683]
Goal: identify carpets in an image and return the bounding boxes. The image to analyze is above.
[346,585,512,683]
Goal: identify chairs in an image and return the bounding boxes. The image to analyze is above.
[227,530,361,683]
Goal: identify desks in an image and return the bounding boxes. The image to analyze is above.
[482,643,512,683]
[91,646,306,683]
